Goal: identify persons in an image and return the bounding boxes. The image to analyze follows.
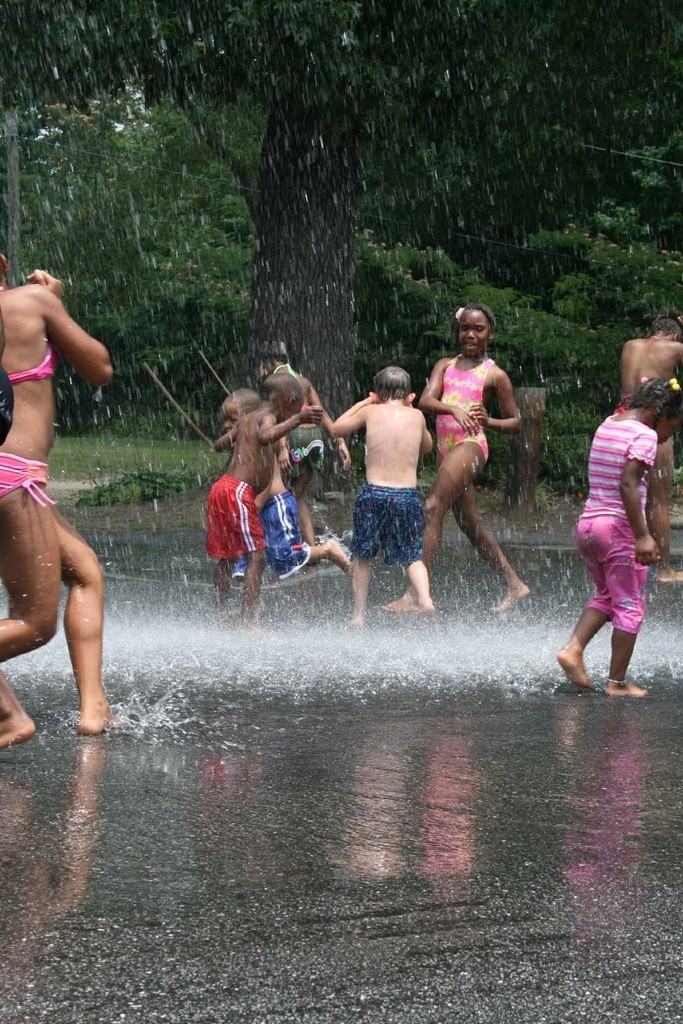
[558,378,682,702]
[616,310,681,582]
[380,304,529,612]
[207,349,437,622]
[0,245,121,755]
